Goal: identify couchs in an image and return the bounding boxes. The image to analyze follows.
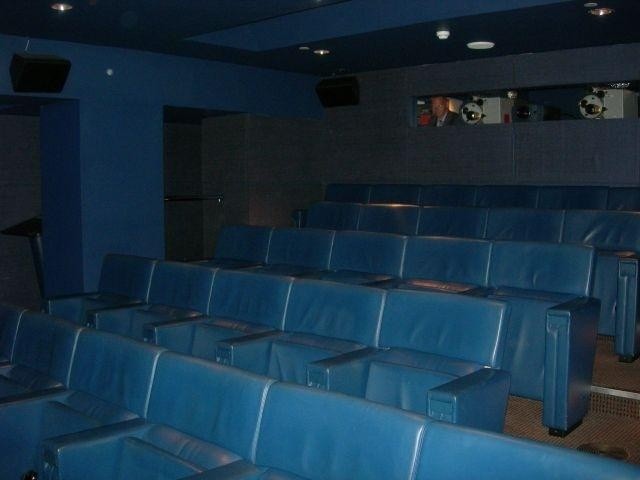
[83,260,220,352]
[359,203,419,236]
[0,328,168,480]
[422,185,478,207]
[563,209,640,362]
[0,310,86,404]
[175,225,275,270]
[418,206,487,239]
[289,231,409,290]
[487,208,564,243]
[412,421,640,480]
[0,301,29,362]
[538,185,609,208]
[178,381,437,480]
[215,278,388,398]
[360,237,494,297]
[307,289,511,436]
[39,352,282,480]
[477,186,540,208]
[291,200,359,230]
[607,187,640,211]
[224,228,336,280]
[376,183,422,206]
[41,255,158,331]
[473,241,602,438]
[325,183,376,204]
[143,270,295,377]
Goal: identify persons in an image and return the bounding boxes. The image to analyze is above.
[428,97,460,127]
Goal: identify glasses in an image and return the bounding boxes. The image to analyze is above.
[430,104,447,109]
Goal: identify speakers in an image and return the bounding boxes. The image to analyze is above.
[315,77,360,106]
[9,52,72,93]
